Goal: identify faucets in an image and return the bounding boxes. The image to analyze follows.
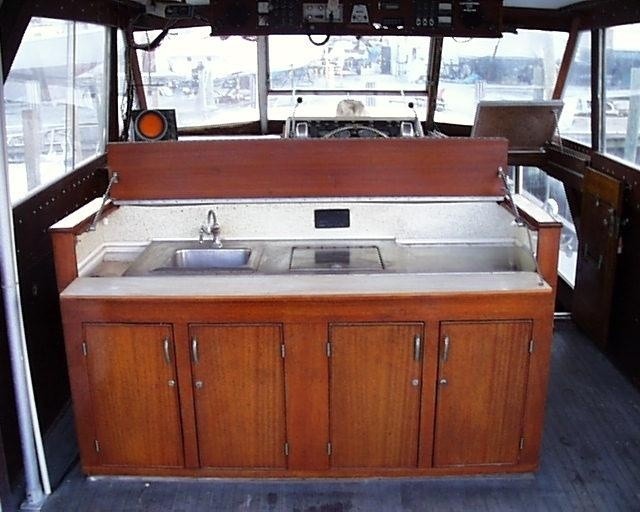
[198,210,223,248]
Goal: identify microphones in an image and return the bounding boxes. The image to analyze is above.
[292,96,303,118]
[408,102,420,121]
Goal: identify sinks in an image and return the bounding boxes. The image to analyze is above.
[148,246,256,271]
